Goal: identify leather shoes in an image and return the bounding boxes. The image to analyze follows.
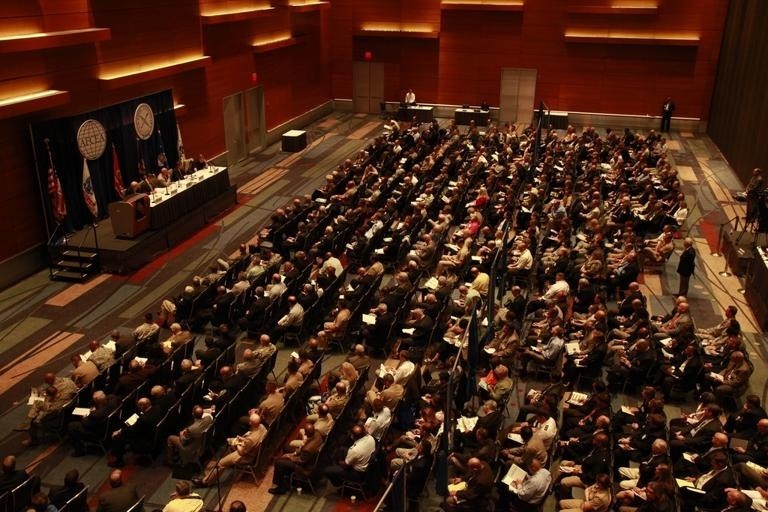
[268,486,288,496]
[192,477,207,486]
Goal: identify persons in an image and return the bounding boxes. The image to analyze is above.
[170,161,186,183]
[481,100,490,111]
[156,167,171,188]
[1,257,640,511]
[190,153,209,173]
[604,282,768,511]
[672,237,696,298]
[405,89,417,106]
[741,167,765,224]
[236,118,689,256]
[125,181,139,195]
[658,96,675,133]
[143,173,156,193]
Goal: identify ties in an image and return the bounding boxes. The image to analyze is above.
[694,469,716,488]
[694,420,707,432]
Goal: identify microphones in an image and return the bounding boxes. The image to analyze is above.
[125,189,139,198]
[142,159,213,203]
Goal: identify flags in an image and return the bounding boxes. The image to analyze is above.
[44,137,67,224]
[82,156,99,220]
[157,130,168,168]
[112,144,126,200]
[177,123,186,162]
[136,138,147,178]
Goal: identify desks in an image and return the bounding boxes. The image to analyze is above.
[397,104,569,131]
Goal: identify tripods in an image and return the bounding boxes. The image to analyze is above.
[736,200,764,252]
